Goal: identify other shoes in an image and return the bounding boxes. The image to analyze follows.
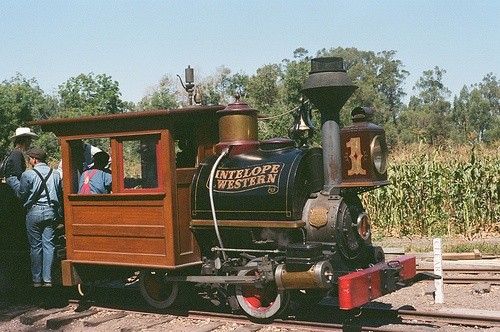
[28,282,53,288]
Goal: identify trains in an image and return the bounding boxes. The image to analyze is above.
[27,57,442,321]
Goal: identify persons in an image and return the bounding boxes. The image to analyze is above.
[5,146,62,287]
[0,127,40,182]
[77,151,113,197]
[55,139,91,219]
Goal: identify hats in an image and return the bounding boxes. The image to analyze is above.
[93,152,109,161]
[9,127,40,140]
[26,148,48,161]
